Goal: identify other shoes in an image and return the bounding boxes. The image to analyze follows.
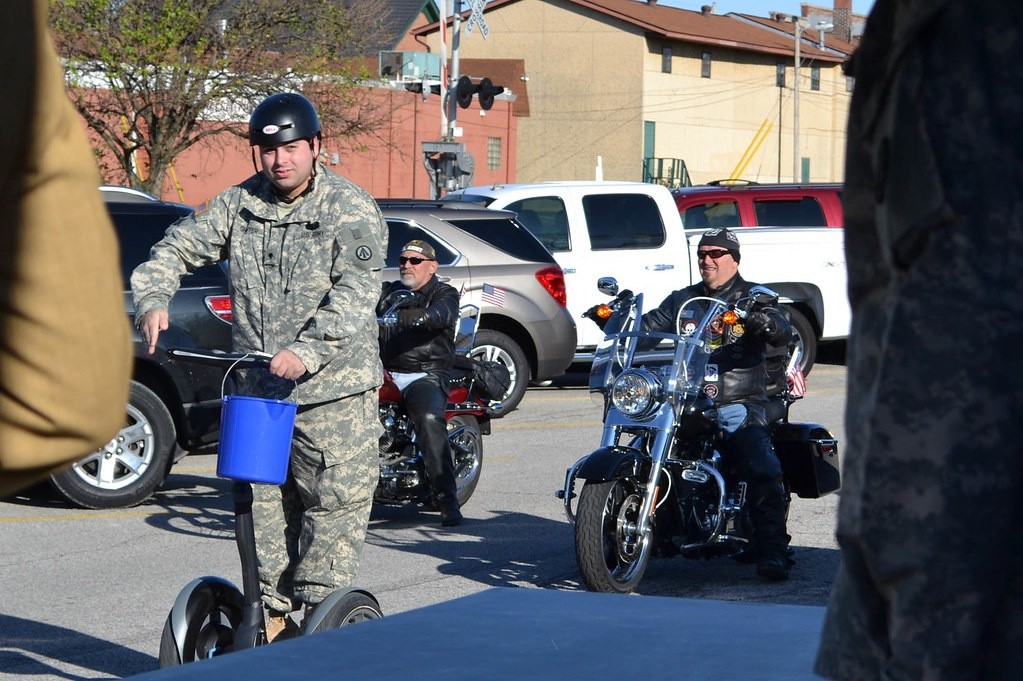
[755,546,797,581]
[265,604,316,644]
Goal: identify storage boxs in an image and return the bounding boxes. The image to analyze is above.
[775,421,842,500]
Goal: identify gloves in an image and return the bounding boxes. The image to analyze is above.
[745,306,769,335]
[396,308,425,329]
[586,304,609,329]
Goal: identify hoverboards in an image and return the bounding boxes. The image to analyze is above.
[158,348,385,673]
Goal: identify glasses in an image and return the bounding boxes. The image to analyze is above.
[697,248,731,259]
[398,255,434,265]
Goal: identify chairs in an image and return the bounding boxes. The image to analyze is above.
[555,211,567,232]
[519,208,544,236]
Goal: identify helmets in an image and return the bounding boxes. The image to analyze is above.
[248,92,322,146]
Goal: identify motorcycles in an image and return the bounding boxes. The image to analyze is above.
[375,291,509,514]
[553,276,841,594]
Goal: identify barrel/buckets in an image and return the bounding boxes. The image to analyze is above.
[215,352,298,484]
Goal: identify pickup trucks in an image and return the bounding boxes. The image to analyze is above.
[441,181,852,370]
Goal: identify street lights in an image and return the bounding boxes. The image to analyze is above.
[793,22,833,180]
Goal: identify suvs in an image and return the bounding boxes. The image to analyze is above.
[40,186,230,510]
[671,179,844,227]
[374,198,578,417]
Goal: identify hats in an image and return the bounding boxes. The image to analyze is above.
[400,240,436,260]
[697,225,740,251]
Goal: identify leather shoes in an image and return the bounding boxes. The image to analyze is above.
[441,497,464,525]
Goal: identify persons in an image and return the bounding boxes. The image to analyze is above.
[374,239,464,527]
[813,0,1023,681]
[587,227,804,575]
[0,1,133,499]
[131,94,389,618]
